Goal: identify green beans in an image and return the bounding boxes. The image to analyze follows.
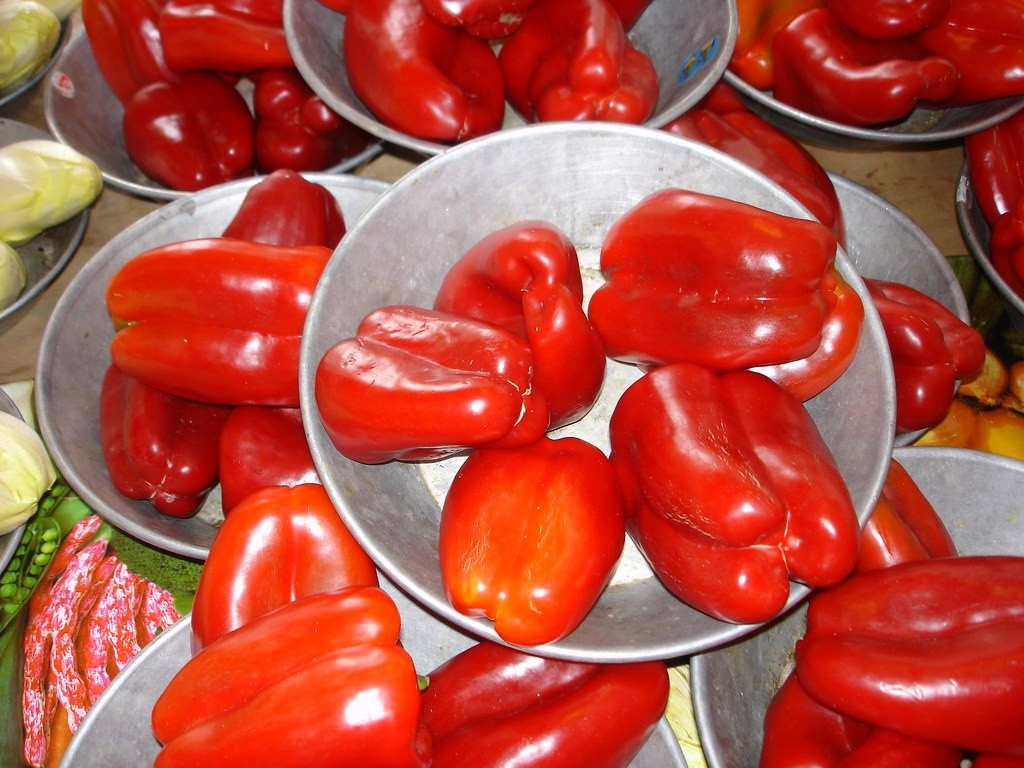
[0,482,111,768]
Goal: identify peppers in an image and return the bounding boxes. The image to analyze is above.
[80,0,1024,768]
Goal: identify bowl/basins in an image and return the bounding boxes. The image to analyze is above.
[0,14,75,113]
[44,24,389,203]
[708,1,1024,148]
[281,0,740,160]
[1,113,1024,768]
